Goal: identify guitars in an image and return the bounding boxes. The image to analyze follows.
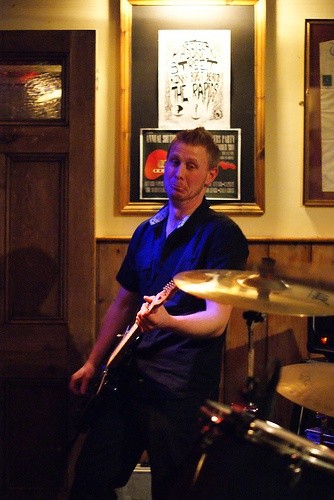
[53,276,178,454]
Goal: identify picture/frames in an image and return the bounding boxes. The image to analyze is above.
[297,17,334,211]
[112,1,272,218]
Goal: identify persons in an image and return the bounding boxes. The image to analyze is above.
[62,123,249,500]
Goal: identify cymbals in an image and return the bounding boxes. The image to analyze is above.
[173,269,334,317]
[268,362,334,417]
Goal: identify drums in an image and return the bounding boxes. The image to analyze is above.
[197,400,334,500]
